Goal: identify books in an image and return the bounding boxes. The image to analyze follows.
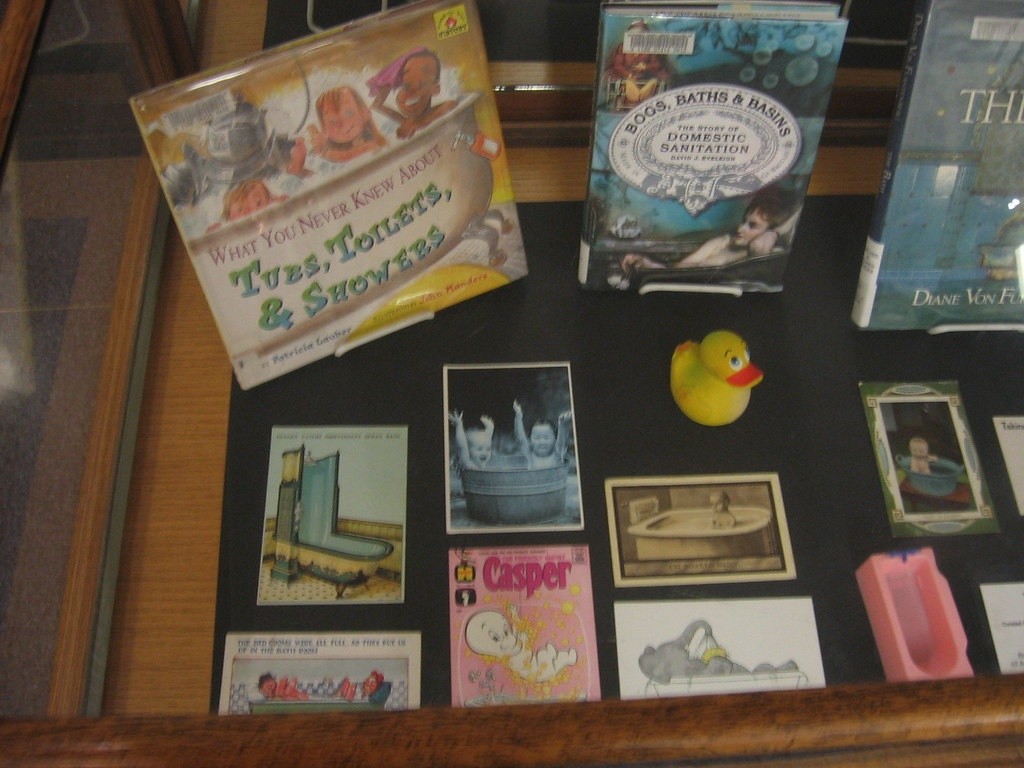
[129,0,530,391]
[579,7,850,292]
[850,0,1024,331]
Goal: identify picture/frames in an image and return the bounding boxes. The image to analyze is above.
[858,380,1001,539]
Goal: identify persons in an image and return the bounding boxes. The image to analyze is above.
[447,396,572,470]
[608,181,801,289]
[708,492,735,531]
